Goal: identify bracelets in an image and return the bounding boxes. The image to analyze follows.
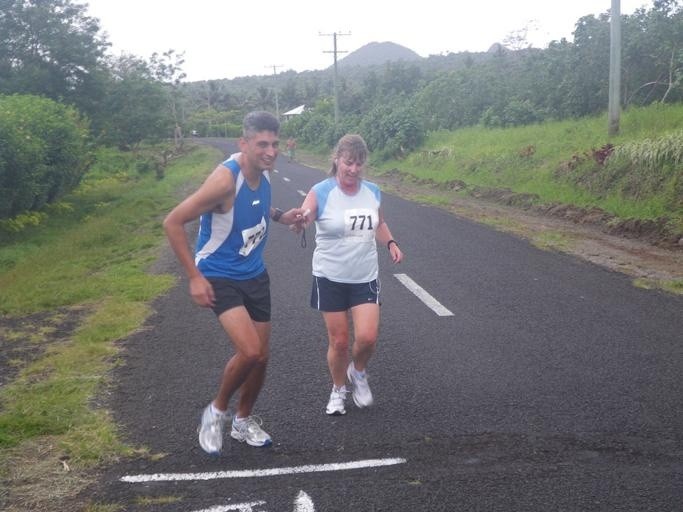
[387,238,398,250]
[271,206,284,223]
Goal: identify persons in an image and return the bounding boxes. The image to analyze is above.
[291,131,404,418]
[285,135,296,165]
[160,110,308,458]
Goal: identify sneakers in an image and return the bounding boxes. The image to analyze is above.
[230,417,271,448]
[198,405,226,455]
[346,363,373,409]
[324,385,347,416]
